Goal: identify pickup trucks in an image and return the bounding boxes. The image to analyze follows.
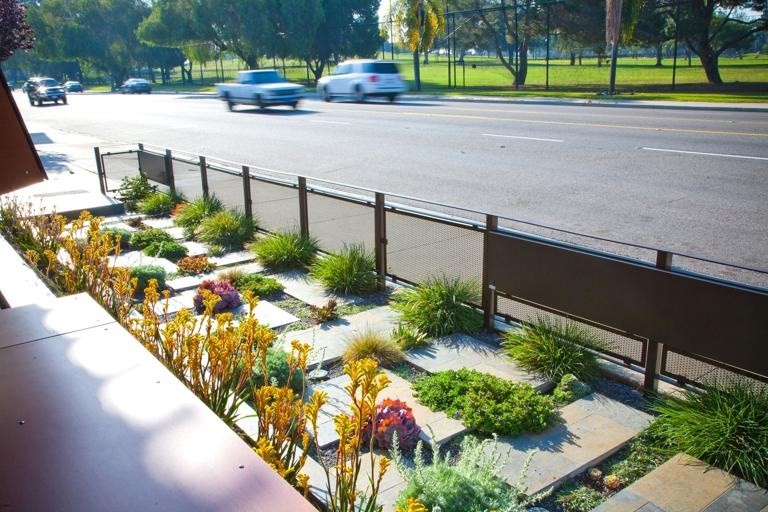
[218,70,305,110]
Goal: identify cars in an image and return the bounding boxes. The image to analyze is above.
[120,78,151,93]
[316,59,408,101]
[6,77,83,105]
